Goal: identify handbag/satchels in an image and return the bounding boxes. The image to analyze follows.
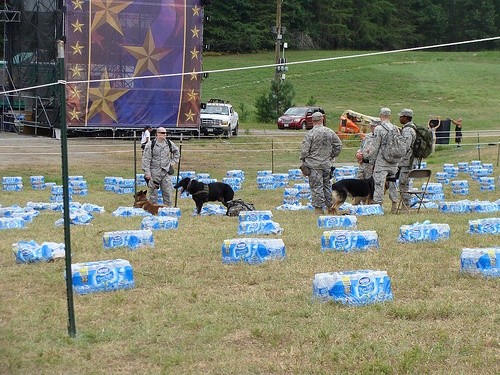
[355,150,363,163]
[403,124,433,159]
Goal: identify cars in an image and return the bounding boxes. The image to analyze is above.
[278,106,327,130]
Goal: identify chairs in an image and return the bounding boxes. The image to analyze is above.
[396,169,431,214]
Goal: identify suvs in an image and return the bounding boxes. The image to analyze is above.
[199,99,239,138]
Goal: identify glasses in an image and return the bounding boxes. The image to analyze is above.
[159,132,167,134]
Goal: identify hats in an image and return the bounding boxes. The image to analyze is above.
[380,108,391,116]
[370,117,382,127]
[398,109,414,117]
[312,112,325,120]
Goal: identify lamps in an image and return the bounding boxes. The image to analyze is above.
[204,43,211,51]
[200,103,206,109]
[204,15,211,23]
[203,73,208,79]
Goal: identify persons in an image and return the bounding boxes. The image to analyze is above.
[141,125,152,150]
[301,112,343,213]
[397,109,417,208]
[355,107,401,214]
[427,118,441,152]
[142,127,179,206]
[450,118,463,147]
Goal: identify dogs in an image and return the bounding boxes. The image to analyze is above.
[173,177,235,215]
[133,190,171,215]
[332,176,388,215]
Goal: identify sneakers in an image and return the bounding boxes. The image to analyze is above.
[391,203,399,214]
[315,207,324,214]
[328,207,331,211]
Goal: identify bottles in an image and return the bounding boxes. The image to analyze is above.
[396,161,500,278]
[0,166,393,305]
